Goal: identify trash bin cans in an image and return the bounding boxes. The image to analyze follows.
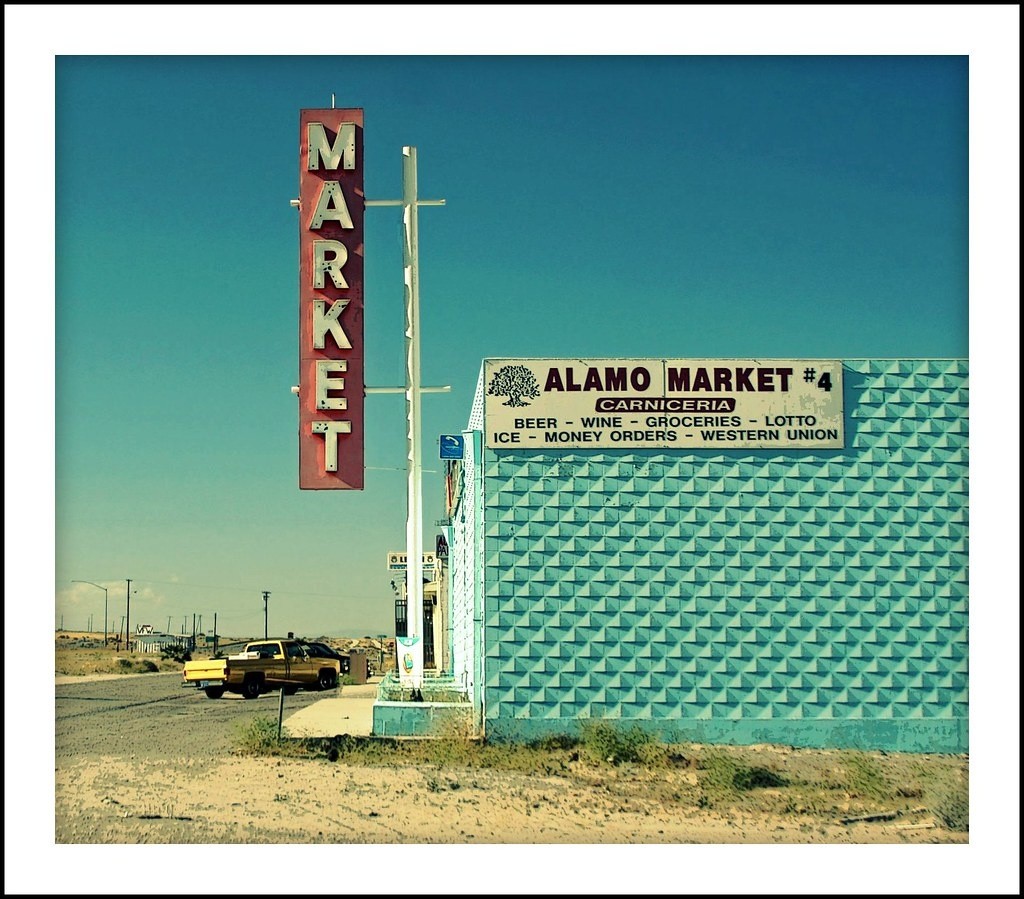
[348,648,367,685]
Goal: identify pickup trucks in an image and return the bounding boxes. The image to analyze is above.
[181,637,343,700]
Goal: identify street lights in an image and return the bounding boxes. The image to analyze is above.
[72,580,108,648]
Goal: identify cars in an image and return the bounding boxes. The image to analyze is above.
[298,642,372,679]
